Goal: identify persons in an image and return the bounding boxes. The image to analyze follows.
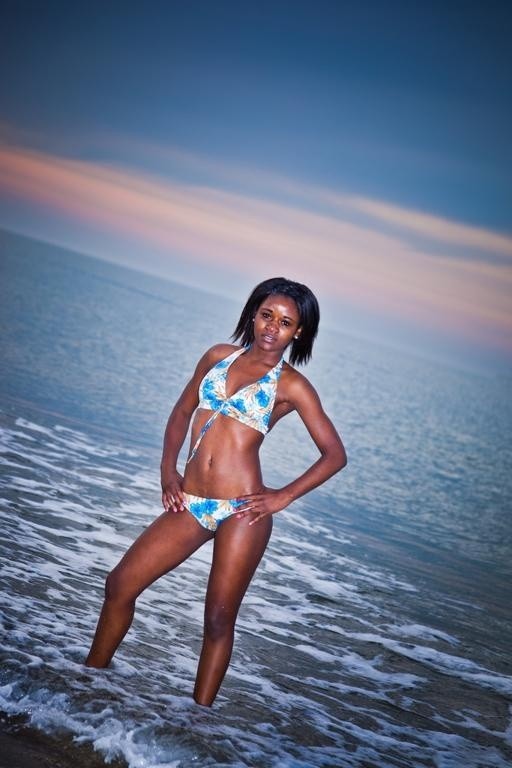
[82,277,347,709]
